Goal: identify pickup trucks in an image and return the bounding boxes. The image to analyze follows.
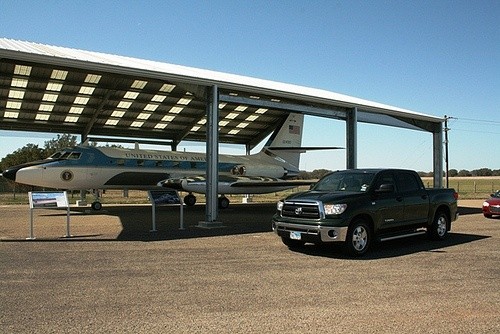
[271,169,459,258]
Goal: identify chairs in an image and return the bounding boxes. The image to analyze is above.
[337,174,358,191]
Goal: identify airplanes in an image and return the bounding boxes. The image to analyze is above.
[0,112,346,211]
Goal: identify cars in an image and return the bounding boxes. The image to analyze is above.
[482,189,500,218]
